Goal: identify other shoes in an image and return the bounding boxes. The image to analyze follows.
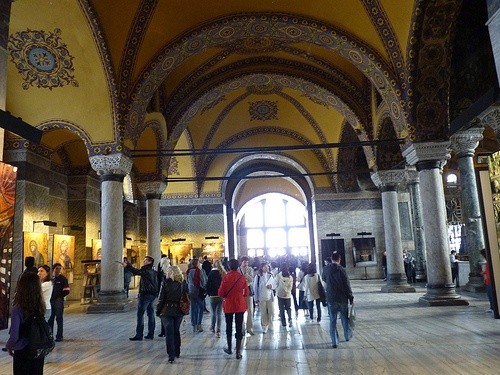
[209,325,215,333]
[55,338,63,341]
[247,329,254,335]
[129,335,143,341]
[288,320,292,327]
[145,334,153,338]
[2,347,8,351]
[224,349,232,354]
[216,331,221,337]
[263,327,268,333]
[192,324,204,332]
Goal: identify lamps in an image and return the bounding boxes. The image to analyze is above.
[135,239,146,243]
[172,237,186,243]
[326,232,341,239]
[63,224,84,232]
[33,220,58,228]
[205,235,219,240]
[356,232,373,238]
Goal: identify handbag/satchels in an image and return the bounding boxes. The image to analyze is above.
[180,280,190,315]
[199,285,208,301]
[317,280,328,307]
[348,304,357,330]
[248,284,255,296]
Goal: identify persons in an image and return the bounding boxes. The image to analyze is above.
[123,254,354,363]
[479,248,493,313]
[2,263,70,375]
[382,249,416,284]
[449,250,459,283]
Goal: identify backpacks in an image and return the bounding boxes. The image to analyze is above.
[21,309,56,359]
